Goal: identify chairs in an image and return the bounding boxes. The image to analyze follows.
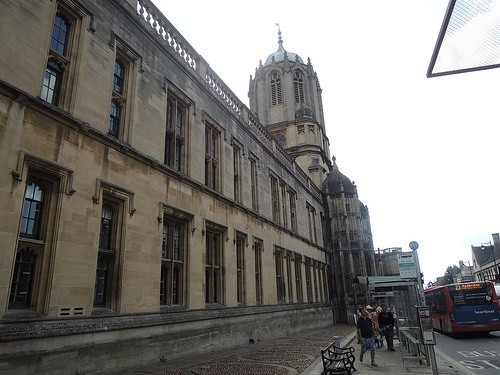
[321,343,358,375]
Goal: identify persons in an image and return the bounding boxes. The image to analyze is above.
[357,308,378,366]
[358,303,395,351]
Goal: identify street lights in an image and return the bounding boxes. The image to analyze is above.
[481,241,498,275]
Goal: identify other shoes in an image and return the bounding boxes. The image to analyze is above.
[387,347,395,350]
[371,361,377,366]
[360,354,363,362]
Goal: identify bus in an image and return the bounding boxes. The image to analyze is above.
[424,281,500,336]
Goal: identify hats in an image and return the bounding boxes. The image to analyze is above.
[366,305,372,309]
